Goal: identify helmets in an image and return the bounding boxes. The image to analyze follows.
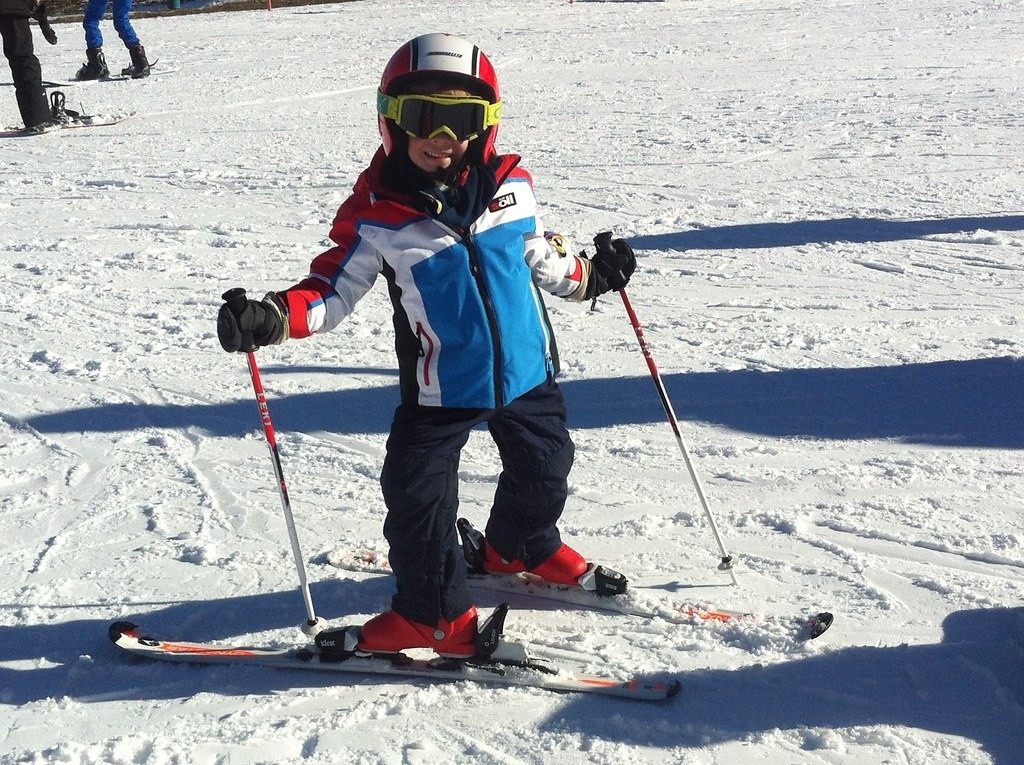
[376,32,501,167]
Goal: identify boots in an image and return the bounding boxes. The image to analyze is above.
[74,49,109,79]
[122,46,150,77]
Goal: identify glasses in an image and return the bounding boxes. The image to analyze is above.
[376,91,502,142]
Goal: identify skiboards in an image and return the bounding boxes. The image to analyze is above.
[0,111,138,138]
[106,545,835,699]
[0,66,177,87]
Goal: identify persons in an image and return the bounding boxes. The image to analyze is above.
[215,31,639,656]
[74,1,150,82]
[0,0,78,126]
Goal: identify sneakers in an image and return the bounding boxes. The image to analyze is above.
[482,539,588,586]
[357,605,478,659]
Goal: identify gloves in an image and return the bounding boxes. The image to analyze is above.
[217,299,281,353]
[590,239,636,298]
[47,28,58,44]
